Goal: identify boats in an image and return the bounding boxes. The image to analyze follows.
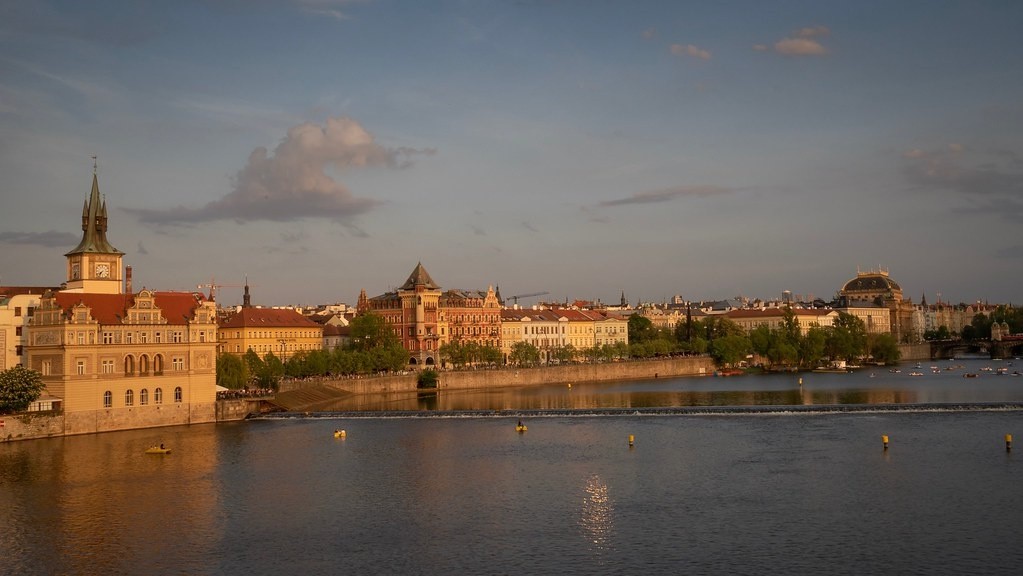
[868,361,1023,378]
[145,443,173,454]
[334,430,346,439]
[515,424,528,432]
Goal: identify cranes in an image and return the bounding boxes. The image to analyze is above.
[506,291,550,304]
[197,276,259,302]
